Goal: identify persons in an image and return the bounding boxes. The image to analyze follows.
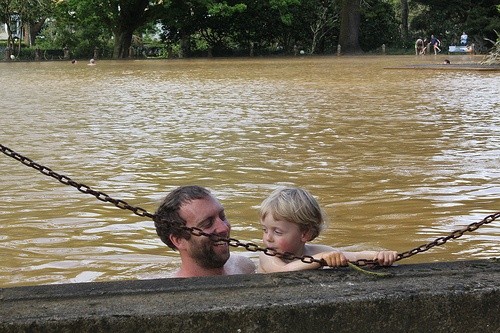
[154,185,256,277]
[443,59,450,64]
[71,59,96,66]
[429,34,441,55]
[461,32,468,55]
[415,38,430,56]
[258,187,398,273]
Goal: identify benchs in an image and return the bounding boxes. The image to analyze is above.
[42,49,65,60]
[449,45,472,55]
